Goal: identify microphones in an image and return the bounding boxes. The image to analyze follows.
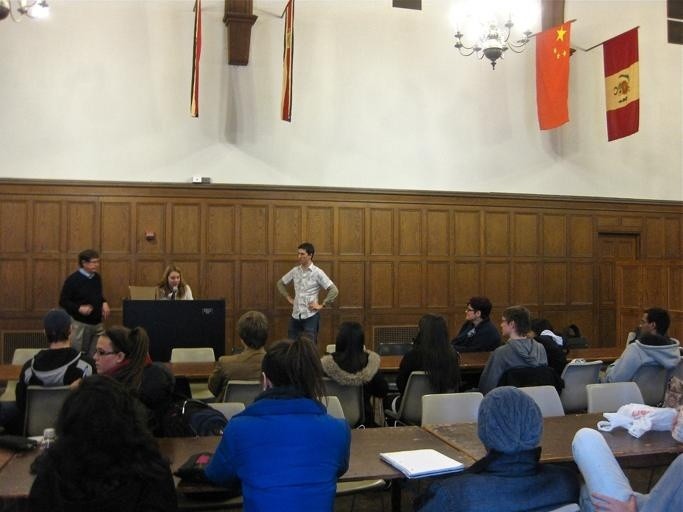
[168,286,179,300]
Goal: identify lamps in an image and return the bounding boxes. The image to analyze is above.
[449,0,541,69]
[1,1,49,23]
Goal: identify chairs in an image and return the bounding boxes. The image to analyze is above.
[421,392,485,426]
[127,287,159,301]
[630,365,667,405]
[516,385,565,418]
[315,396,345,420]
[322,344,367,380]
[0,348,51,402]
[585,382,646,413]
[561,361,602,410]
[23,387,72,436]
[627,332,638,347]
[335,478,392,511]
[383,371,436,423]
[319,375,365,428]
[206,403,246,422]
[222,379,260,408]
[169,348,217,399]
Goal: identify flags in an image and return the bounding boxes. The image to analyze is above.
[533,20,570,131]
[601,25,641,142]
[188,0,203,118]
[279,0,296,122]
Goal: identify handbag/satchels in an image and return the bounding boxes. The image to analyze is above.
[496,365,565,397]
[560,324,587,349]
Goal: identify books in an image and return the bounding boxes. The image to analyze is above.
[378,448,465,480]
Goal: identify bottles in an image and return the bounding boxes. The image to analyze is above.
[38,428,57,452]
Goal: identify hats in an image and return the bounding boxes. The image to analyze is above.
[476,385,545,453]
[43,308,74,330]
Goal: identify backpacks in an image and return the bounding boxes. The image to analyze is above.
[549,351,567,373]
[176,398,229,437]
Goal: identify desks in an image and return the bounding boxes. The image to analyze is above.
[422,403,682,464]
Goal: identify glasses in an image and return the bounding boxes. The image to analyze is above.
[466,307,476,312]
[93,347,119,356]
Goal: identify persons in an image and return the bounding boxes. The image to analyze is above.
[208,310,269,402]
[318,320,389,426]
[158,264,194,300]
[601,307,682,383]
[379,313,462,424]
[475,304,548,394]
[26,373,179,512]
[569,426,683,511]
[2,307,93,436]
[527,316,569,377]
[203,337,352,512]
[449,295,501,354]
[277,242,339,345]
[93,324,176,438]
[60,248,111,359]
[415,384,581,512]
